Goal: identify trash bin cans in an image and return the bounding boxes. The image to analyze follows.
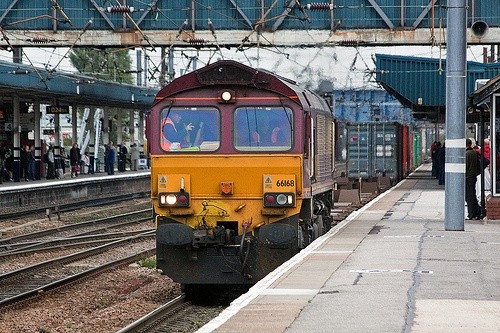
[138,154,147,169]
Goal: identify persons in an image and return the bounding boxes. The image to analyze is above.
[1,141,145,181]
[430,139,491,192]
[161,111,286,151]
[465,140,482,220]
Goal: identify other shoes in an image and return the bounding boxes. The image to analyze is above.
[465,216,482,221]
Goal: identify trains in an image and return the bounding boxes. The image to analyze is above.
[149,59,347,292]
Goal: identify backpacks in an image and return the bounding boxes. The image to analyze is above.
[43,151,50,164]
